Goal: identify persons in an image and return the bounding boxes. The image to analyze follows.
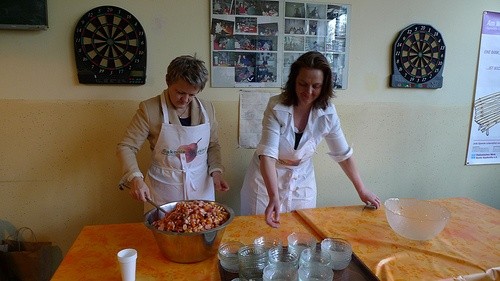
[213,0,348,88]
[117,55,231,222]
[240,51,382,229]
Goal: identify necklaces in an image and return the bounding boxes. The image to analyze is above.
[174,105,188,118]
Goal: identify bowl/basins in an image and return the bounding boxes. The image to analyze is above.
[217,232,352,281]
[143,199,235,264]
[383,197,451,241]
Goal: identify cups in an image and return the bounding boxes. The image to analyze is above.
[116,248,138,281]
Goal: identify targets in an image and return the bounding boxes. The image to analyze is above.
[72,5,148,86]
[391,22,445,89]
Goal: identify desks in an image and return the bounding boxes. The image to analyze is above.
[49,197,500,281]
